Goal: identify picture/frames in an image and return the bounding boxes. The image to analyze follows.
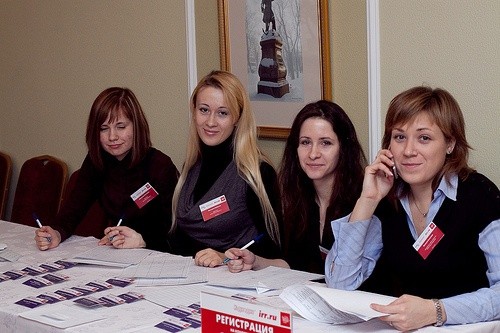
[216,0,334,141]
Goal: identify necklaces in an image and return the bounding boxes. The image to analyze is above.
[410,188,428,217]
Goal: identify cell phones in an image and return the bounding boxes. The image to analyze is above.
[391,158,398,179]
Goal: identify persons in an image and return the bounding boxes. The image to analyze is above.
[323,85,500,331]
[225,99,369,284]
[35,88,180,253]
[104,70,281,268]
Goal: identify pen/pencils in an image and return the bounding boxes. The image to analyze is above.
[109,218,123,241]
[35,218,51,242]
[220,240,255,265]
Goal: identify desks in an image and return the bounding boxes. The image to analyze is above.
[0,218,500,333]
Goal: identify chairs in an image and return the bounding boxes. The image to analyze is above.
[58,169,113,239]
[9,155,67,230]
[0,151,14,220]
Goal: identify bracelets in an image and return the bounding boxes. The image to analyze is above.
[433,298,443,327]
